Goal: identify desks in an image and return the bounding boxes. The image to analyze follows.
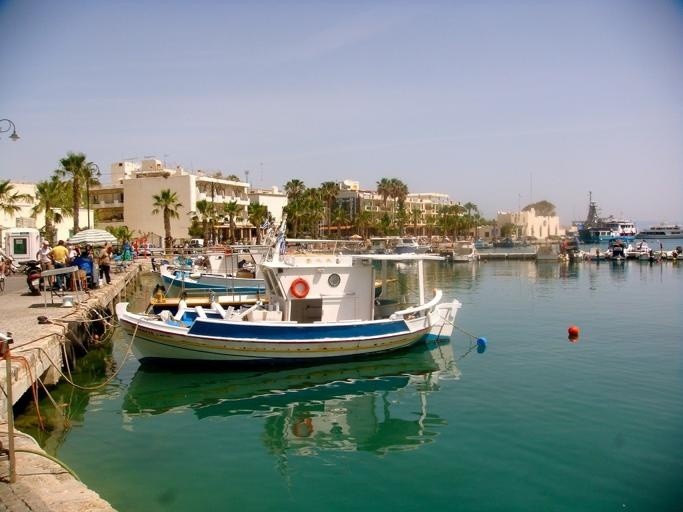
[41,265,83,308]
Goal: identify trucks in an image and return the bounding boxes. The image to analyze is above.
[6,226,41,263]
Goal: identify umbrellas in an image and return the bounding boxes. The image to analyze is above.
[68,228,118,247]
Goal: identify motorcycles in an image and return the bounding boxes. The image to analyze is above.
[18,260,45,294]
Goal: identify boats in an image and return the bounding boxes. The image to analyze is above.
[572,191,683,240]
[568,241,683,260]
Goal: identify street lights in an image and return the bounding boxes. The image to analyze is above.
[1,119,20,143]
[87,162,101,228]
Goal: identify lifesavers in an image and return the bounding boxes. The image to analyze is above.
[291,279,310,299]
[225,247,233,257]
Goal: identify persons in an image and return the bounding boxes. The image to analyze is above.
[35,239,113,293]
[130,242,136,252]
[0,261,8,280]
[121,241,131,261]
